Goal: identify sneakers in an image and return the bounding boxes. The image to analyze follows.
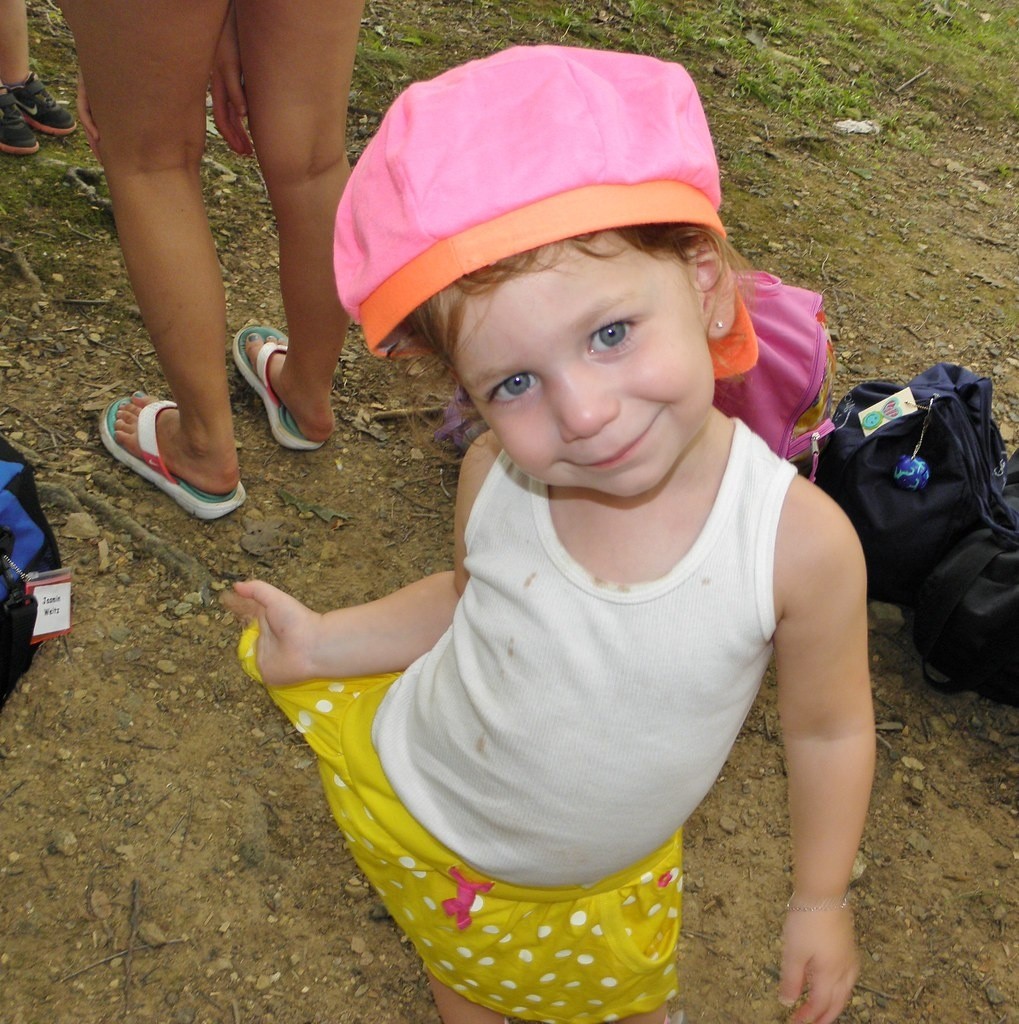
[0,71,77,154]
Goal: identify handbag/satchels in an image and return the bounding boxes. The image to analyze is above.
[0,434,73,714]
[801,362,1019,708]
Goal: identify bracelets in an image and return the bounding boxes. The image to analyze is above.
[783,889,848,911]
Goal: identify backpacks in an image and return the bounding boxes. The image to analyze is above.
[713,270,835,482]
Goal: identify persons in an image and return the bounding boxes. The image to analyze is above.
[0,0,77,155]
[63,0,364,521]
[221,50,876,1023]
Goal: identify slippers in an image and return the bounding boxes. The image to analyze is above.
[99,396,246,519]
[232,324,325,449]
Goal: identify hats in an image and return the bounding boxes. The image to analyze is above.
[333,44,759,381]
